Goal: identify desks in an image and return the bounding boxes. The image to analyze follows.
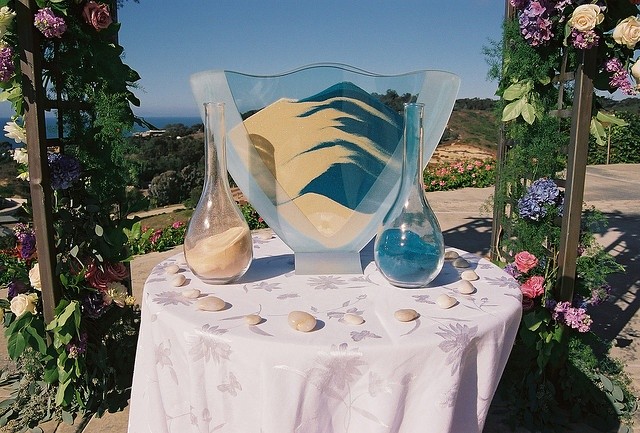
[139,230,523,432]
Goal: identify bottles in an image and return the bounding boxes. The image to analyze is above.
[374,102,445,288]
[183,102,253,285]
